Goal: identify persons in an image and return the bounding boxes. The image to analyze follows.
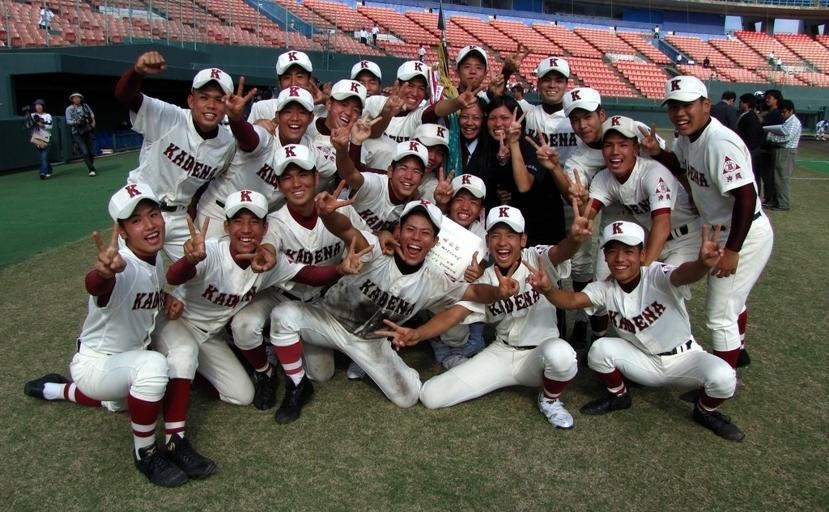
[27,99,53,179]
[38,2,55,30]
[360,27,368,46]
[372,24,379,46]
[108,45,703,427]
[710,90,801,210]
[22,183,190,486]
[815,120,829,141]
[639,76,774,402]
[66,92,97,175]
[654,26,660,40]
[149,190,376,477]
[522,220,745,442]
[703,56,710,68]
[769,51,781,71]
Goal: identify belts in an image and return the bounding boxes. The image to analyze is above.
[215,199,224,208]
[665,223,688,243]
[271,284,315,303]
[160,197,184,213]
[657,341,692,355]
[502,338,536,350]
[712,212,761,232]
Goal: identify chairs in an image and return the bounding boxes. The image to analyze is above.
[0,0,829,101]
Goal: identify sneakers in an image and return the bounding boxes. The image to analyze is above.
[88,171,96,176]
[690,402,745,441]
[252,364,278,411]
[133,433,216,487]
[538,388,573,428]
[274,375,314,424]
[763,199,789,210]
[348,361,366,380]
[24,374,69,400]
[442,354,470,370]
[40,173,54,178]
[579,390,633,414]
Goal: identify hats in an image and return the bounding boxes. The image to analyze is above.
[399,200,441,233]
[455,44,489,70]
[225,188,271,220]
[273,49,451,176]
[486,204,524,234]
[108,183,159,224]
[452,173,486,200]
[70,91,83,101]
[33,99,44,105]
[190,68,235,97]
[537,57,708,139]
[600,221,646,251]
